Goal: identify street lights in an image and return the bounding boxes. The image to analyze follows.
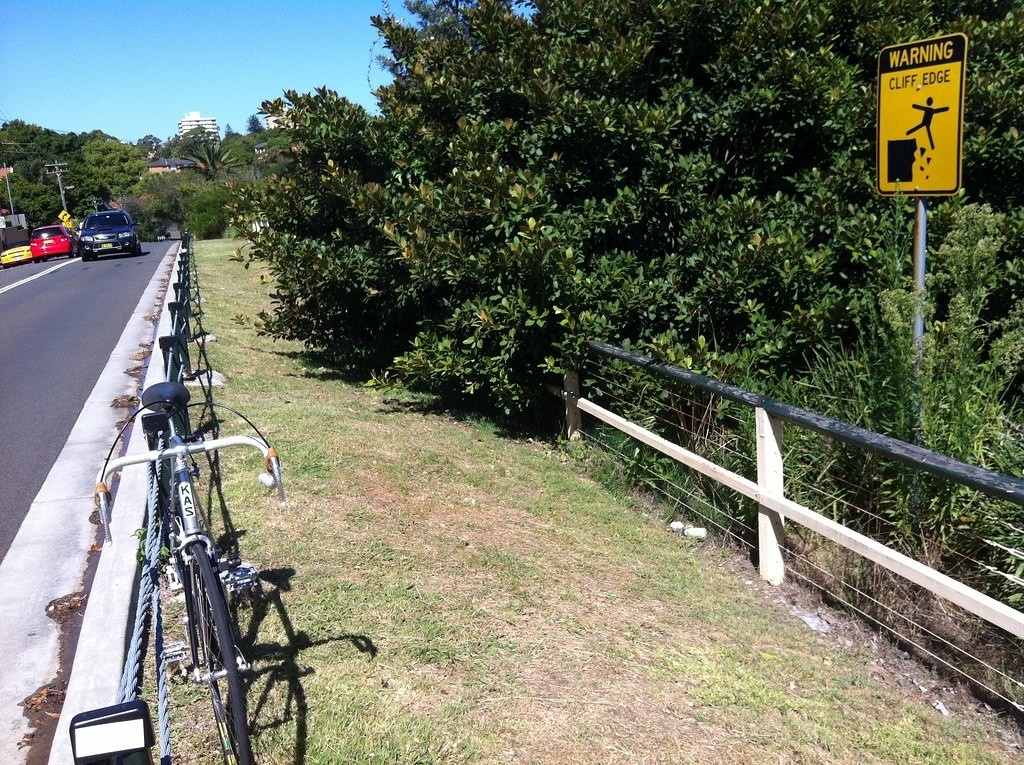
[62,186,75,230]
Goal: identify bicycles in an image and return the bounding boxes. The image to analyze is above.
[94,382,286,765]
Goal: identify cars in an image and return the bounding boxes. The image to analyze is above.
[0,241,33,268]
[31,225,79,263]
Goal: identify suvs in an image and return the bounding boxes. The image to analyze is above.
[79,210,141,262]
